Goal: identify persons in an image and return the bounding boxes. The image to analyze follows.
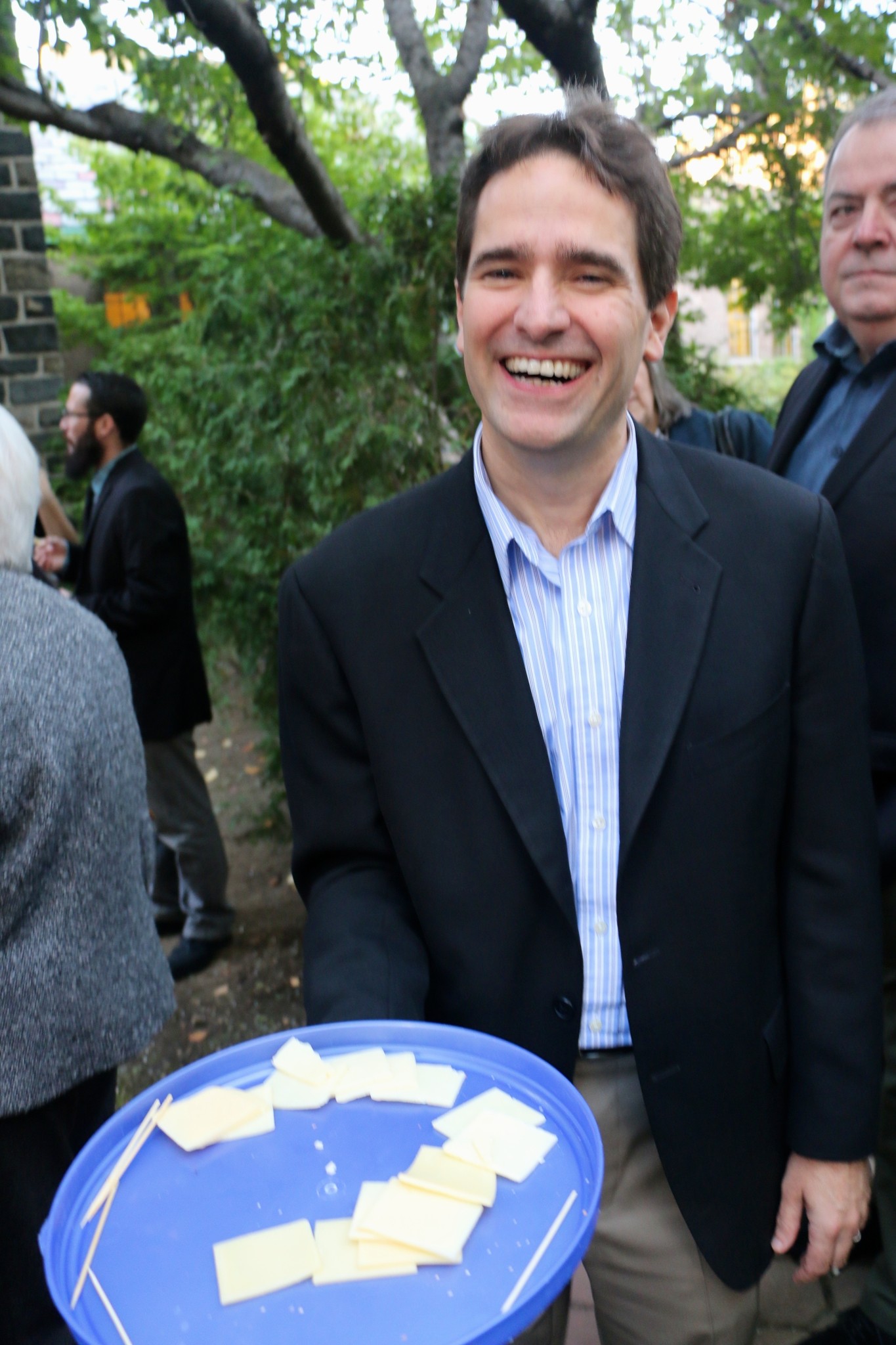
[0,341,232,1345]
[281,92,896,1345]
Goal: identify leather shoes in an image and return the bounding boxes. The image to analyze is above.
[166,934,232,981]
[150,913,186,935]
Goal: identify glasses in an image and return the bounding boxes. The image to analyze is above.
[61,407,92,420]
